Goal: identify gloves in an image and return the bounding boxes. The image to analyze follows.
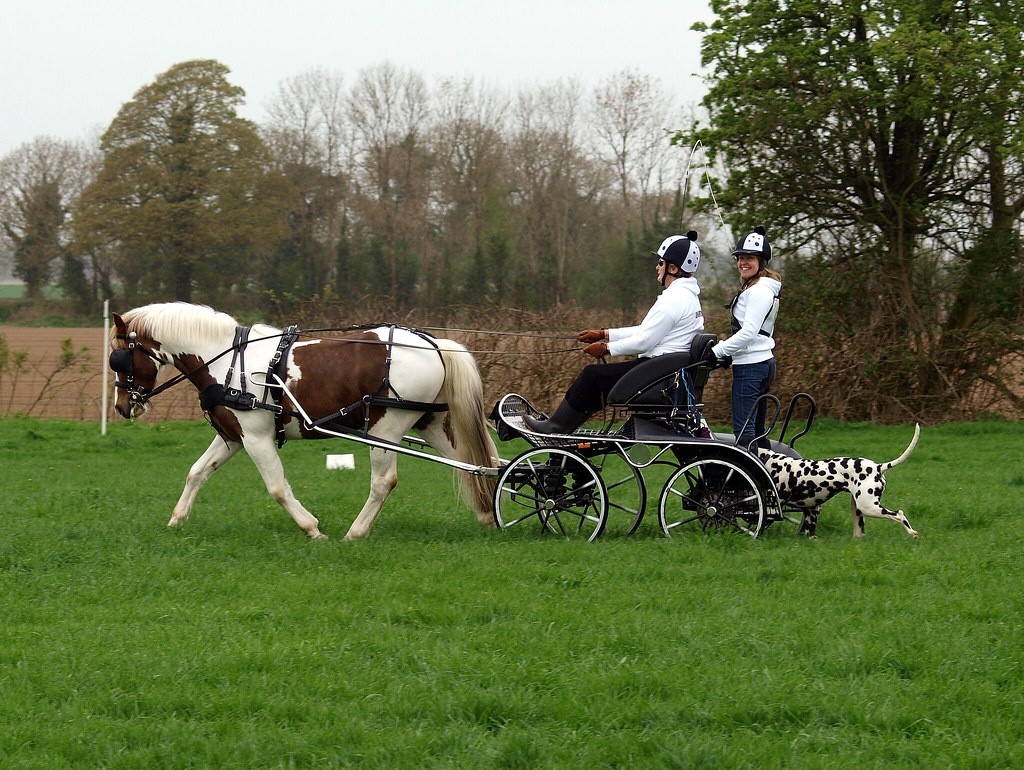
[583,342,607,359]
[577,329,606,344]
[705,350,718,370]
[721,355,732,369]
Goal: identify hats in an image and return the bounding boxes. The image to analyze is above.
[731,226,771,261]
[651,231,701,277]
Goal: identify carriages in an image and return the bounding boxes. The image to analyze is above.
[108,302,816,542]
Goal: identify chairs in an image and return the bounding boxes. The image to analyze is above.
[609,334,718,413]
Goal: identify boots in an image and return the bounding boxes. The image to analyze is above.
[522,397,594,442]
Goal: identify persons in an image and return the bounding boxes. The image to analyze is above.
[521,229,707,435]
[705,232,782,457]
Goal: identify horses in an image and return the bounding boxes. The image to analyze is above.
[109,301,501,541]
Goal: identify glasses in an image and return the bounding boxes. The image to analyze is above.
[658,258,664,266]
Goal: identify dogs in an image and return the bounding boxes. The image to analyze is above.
[759,422,920,538]
